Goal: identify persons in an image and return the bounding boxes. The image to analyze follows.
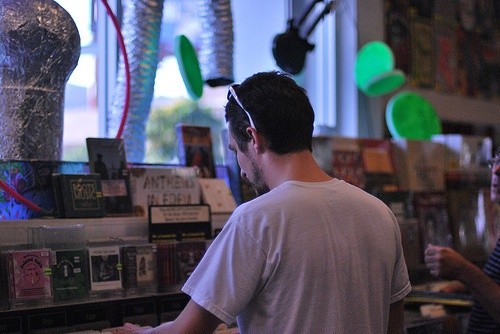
[109,71,412,334]
[425,150,500,334]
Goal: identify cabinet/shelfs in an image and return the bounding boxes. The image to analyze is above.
[0,160,500,334]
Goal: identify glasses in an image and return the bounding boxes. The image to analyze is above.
[488,158,500,167]
[227,84,257,132]
